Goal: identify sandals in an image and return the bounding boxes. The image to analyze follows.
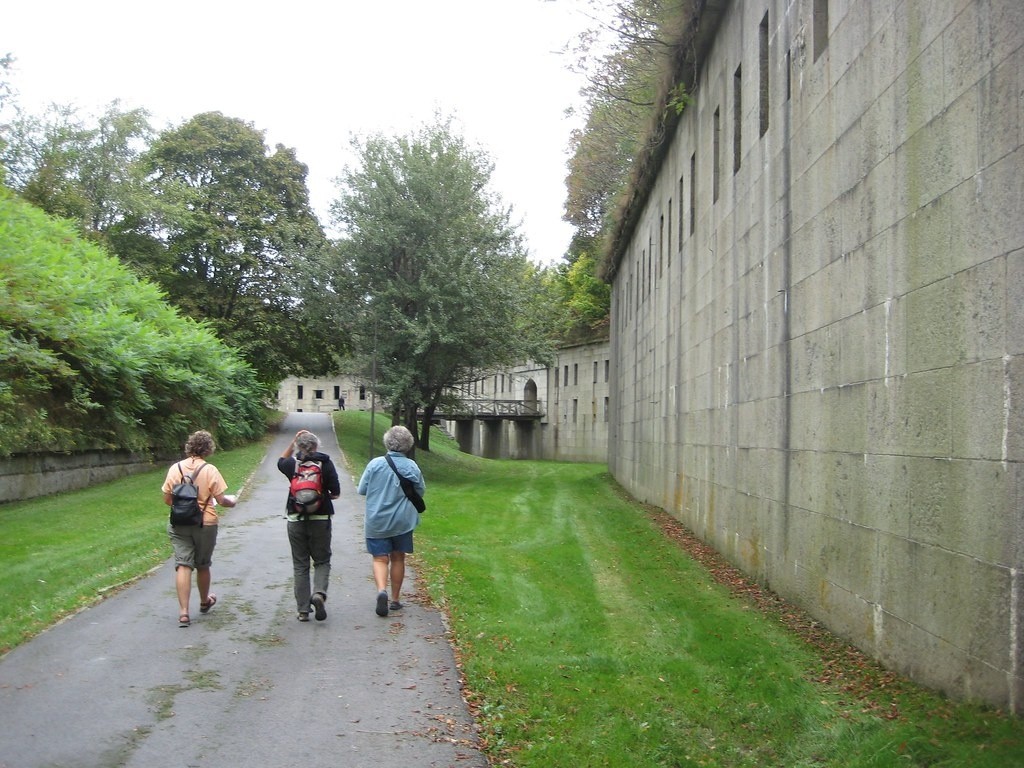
[199,594,216,612]
[178,614,190,626]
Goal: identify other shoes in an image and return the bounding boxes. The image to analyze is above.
[312,594,327,620]
[297,611,309,620]
[375,590,389,616]
[390,600,403,610]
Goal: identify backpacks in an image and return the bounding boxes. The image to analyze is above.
[170,463,213,527]
[288,456,326,522]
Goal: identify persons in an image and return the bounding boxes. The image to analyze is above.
[277,430,340,622]
[338,396,345,410]
[162,429,239,627]
[357,425,425,617]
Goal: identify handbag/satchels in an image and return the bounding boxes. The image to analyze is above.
[399,477,425,513]
